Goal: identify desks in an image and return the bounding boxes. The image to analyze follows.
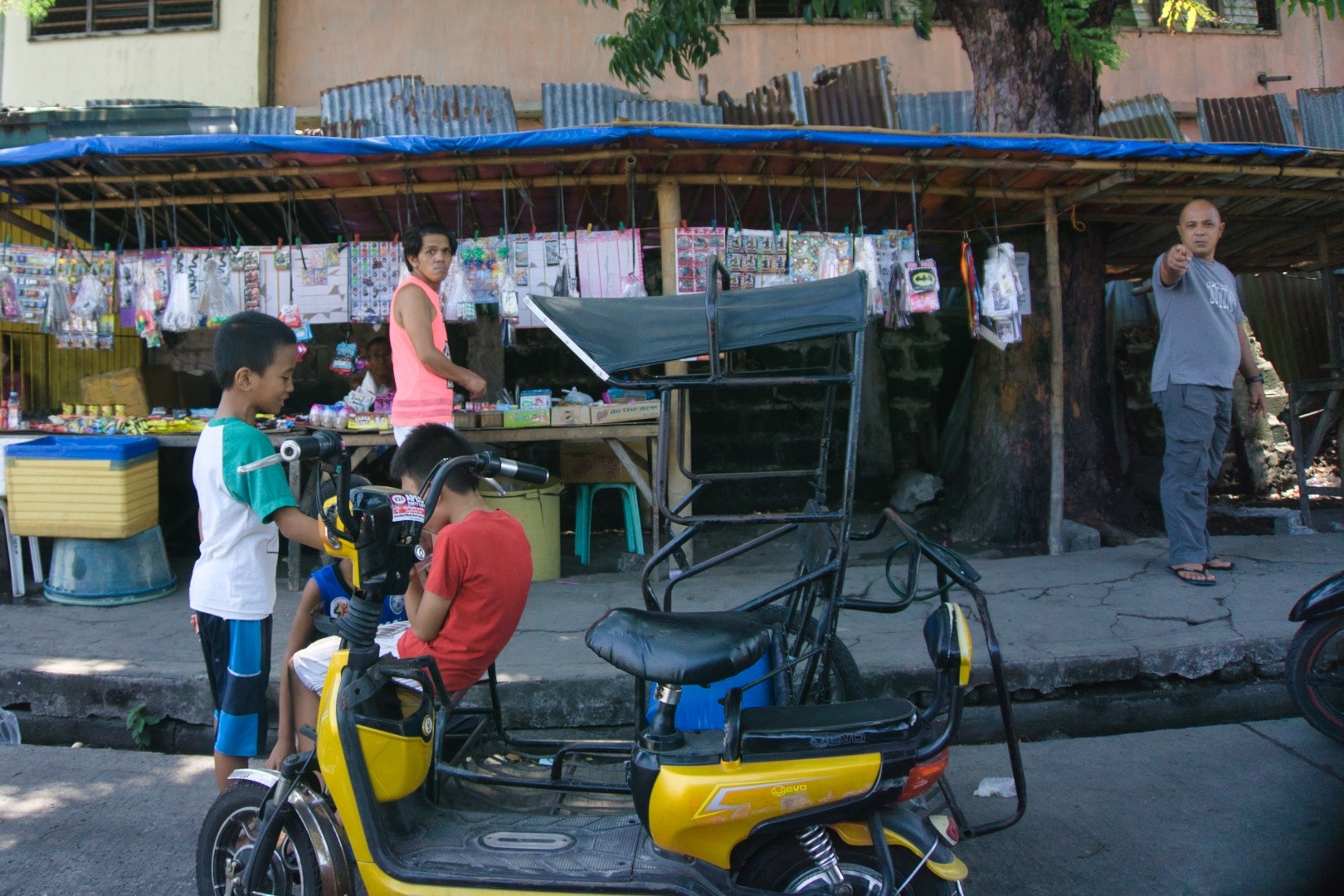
[320,423,662,582]
[0,418,311,588]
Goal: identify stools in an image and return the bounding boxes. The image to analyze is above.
[574,479,642,564]
[0,500,44,597]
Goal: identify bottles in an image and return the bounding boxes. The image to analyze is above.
[0,400,8,430]
[8,392,22,430]
[308,403,355,429]
[62,401,127,416]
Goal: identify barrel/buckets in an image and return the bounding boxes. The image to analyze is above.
[476,476,566,582]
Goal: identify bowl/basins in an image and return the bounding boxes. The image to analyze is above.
[44,525,178,605]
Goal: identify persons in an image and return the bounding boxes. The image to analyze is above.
[350,337,395,411]
[265,423,533,856]
[1152,199,1267,585]
[190,311,324,853]
[389,220,487,448]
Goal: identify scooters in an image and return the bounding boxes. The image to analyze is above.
[196,257,1028,896]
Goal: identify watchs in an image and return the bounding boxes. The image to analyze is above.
[1246,373,1265,385]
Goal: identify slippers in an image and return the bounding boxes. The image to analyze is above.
[1167,561,1216,586]
[1203,556,1236,570]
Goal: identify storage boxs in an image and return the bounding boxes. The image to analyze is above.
[348,387,658,479]
[8,436,159,538]
